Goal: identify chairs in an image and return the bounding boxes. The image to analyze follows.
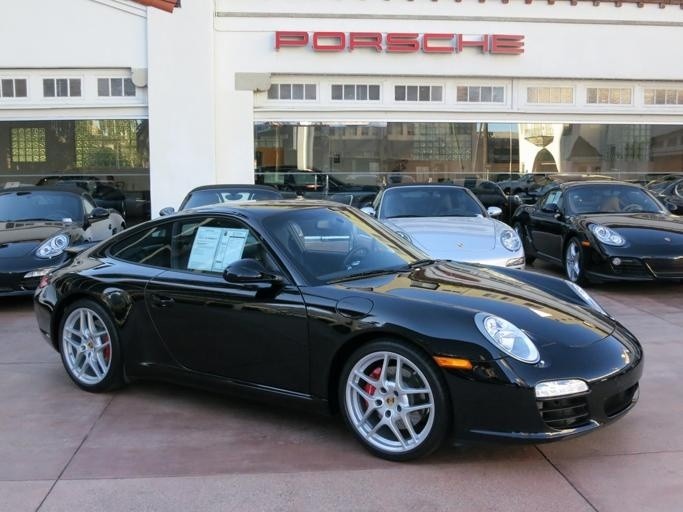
[261,217,305,276]
